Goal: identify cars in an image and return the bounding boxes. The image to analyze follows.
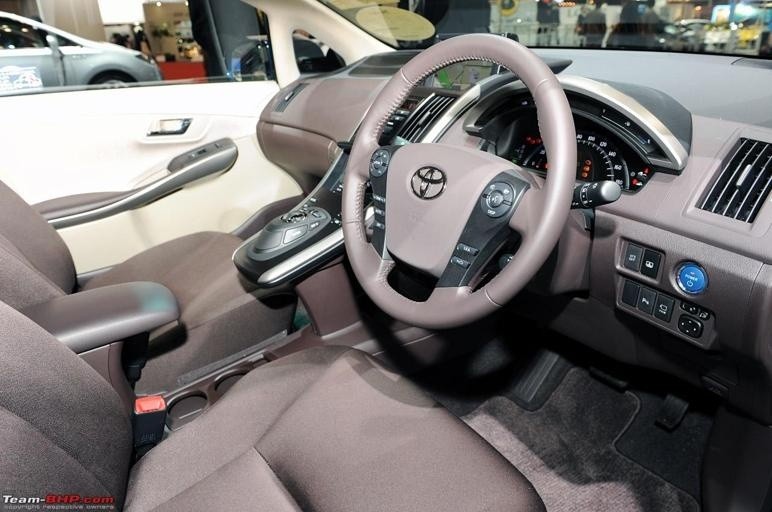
[0,9,165,90]
[649,14,725,52]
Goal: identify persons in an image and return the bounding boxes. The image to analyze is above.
[534,1,666,49]
